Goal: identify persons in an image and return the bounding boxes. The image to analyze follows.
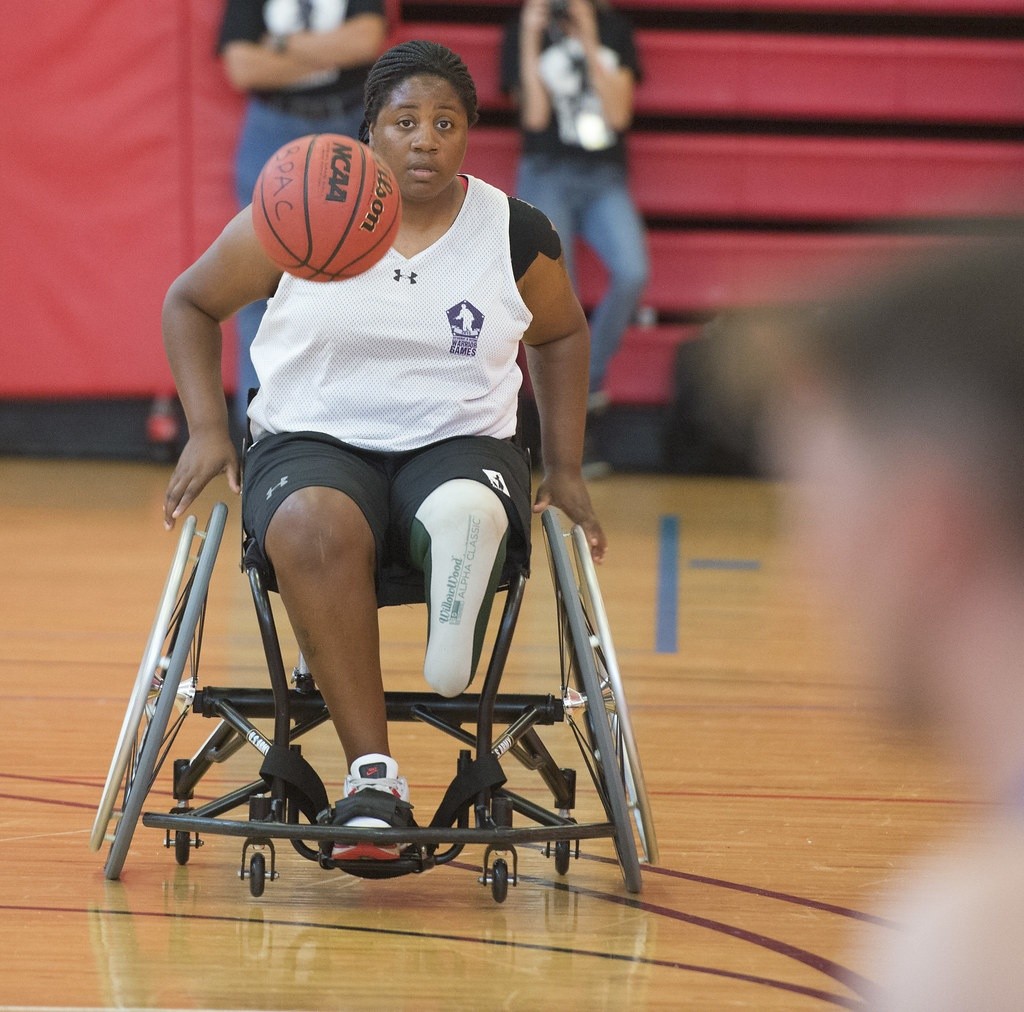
[163,44,606,861]
[215,0,387,445]
[500,0,648,417]
[748,248,1024,1012]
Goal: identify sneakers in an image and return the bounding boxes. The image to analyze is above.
[330,754,417,861]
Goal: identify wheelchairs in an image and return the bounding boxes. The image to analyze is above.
[91,387,659,895]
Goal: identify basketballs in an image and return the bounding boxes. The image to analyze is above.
[251,130,403,284]
